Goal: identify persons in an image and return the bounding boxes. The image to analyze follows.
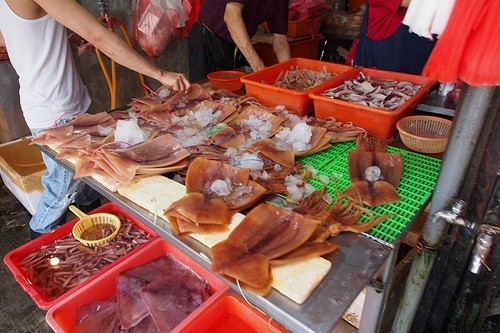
[345,0,437,75]
[188,0,291,84]
[0,0,192,241]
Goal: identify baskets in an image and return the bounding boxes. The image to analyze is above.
[69,205,121,247]
[207,70,247,92]
[396,115,454,152]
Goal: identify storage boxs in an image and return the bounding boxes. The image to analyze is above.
[45,236,230,333]
[179,293,290,333]
[1,135,48,215]
[240,57,356,116]
[2,201,159,311]
[308,67,437,140]
[287,17,321,37]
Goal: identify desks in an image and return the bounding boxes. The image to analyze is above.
[253,35,324,66]
